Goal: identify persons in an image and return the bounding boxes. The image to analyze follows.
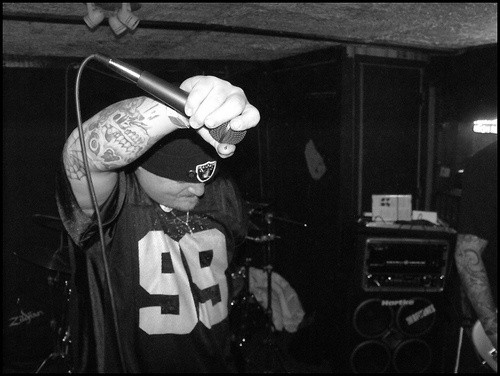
[53,75,261,374]
[445,139,500,374]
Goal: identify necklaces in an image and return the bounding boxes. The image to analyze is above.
[171,207,197,244]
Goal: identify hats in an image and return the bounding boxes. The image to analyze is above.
[138,129,223,183]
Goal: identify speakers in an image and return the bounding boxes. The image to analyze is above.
[344,208,457,376]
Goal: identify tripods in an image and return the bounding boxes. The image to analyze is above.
[36,216,300,376]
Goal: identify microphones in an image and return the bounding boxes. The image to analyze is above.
[94,53,247,144]
